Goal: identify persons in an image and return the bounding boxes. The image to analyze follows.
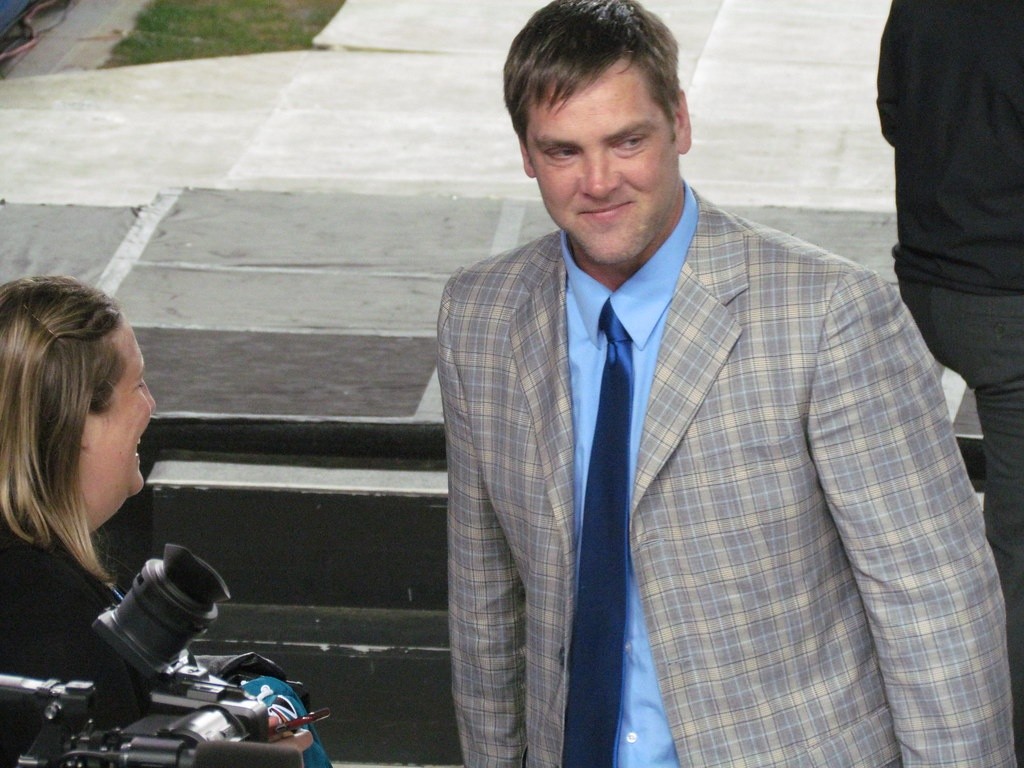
[436,0,1016,767]
[877,0,1024,767]
[0,273,316,767]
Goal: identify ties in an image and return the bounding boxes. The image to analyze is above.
[562,297,634,768]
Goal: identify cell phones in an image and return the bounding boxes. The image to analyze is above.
[274,707,331,734]
